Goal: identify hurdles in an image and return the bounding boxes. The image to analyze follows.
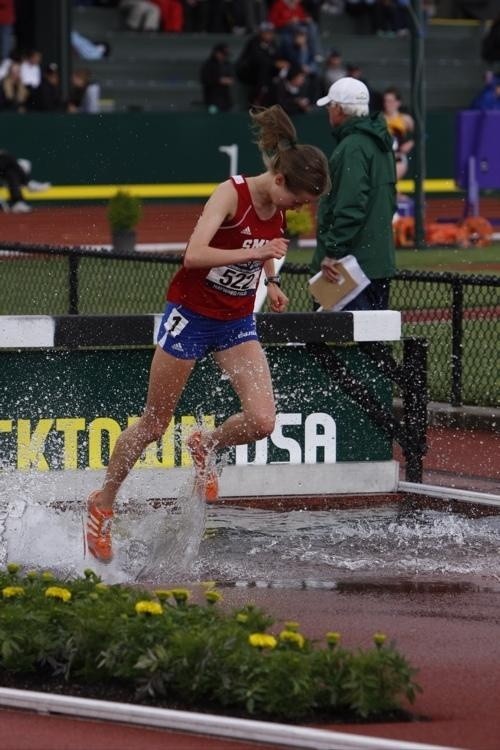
[0,309,427,504]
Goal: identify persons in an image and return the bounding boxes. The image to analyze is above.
[84,104,331,565]
[308,77,397,312]
[0,155,50,215]
[472,12,500,112]
[199,0,434,181]
[2,1,161,116]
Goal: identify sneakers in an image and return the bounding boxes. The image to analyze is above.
[188,431,219,504]
[84,489,116,564]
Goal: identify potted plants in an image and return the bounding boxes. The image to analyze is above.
[286,204,311,246]
[107,188,141,251]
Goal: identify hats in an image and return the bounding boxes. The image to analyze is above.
[316,76,370,106]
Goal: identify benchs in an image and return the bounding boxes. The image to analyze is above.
[0,0,500,111]
